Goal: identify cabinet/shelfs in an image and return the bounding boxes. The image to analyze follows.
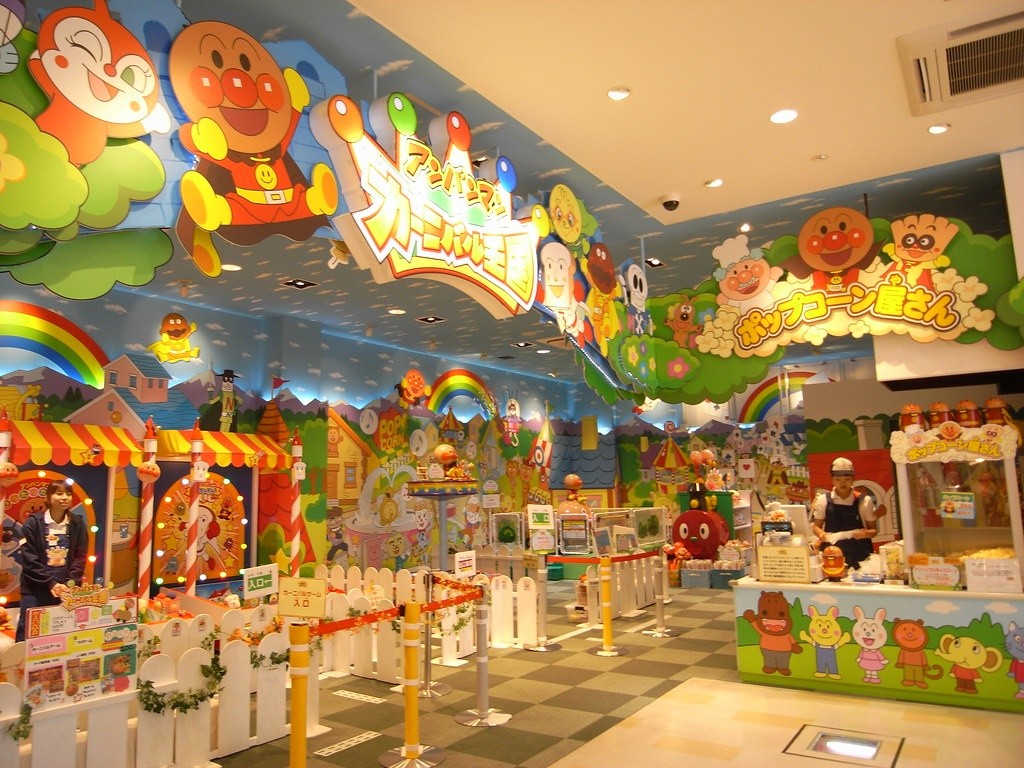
[721,489,754,576]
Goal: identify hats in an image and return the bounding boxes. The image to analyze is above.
[830,457,854,475]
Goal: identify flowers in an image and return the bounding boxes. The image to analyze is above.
[135,616,291,717]
[303,575,483,654]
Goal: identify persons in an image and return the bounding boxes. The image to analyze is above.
[813,457,878,569]
[14,479,90,642]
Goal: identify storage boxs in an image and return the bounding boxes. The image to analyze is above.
[711,570,745,589]
[680,568,711,588]
[565,584,599,622]
[548,566,563,580]
